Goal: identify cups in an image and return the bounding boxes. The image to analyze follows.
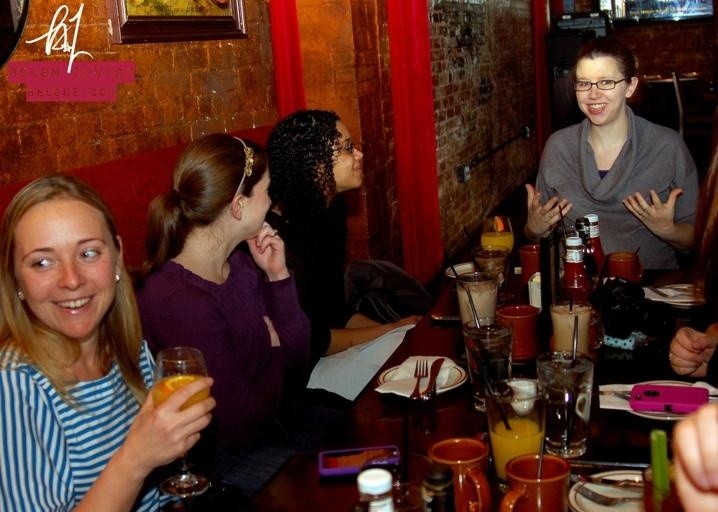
[644,464,684,511]
[428,299,594,512]
[590,251,645,339]
[519,244,541,285]
[455,214,513,324]
[527,271,542,309]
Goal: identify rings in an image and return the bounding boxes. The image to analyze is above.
[270,226,280,238]
[667,349,676,361]
[638,209,648,217]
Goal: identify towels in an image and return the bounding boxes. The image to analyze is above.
[374,354,457,397]
[597,381,717,411]
[641,287,705,302]
[305,324,418,402]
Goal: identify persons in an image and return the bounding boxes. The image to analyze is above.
[0,172,247,512]
[671,399,718,511]
[524,38,701,271]
[240,110,428,417]
[668,125,717,389]
[139,132,311,492]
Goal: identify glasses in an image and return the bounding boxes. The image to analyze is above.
[331,141,356,154]
[572,76,632,91]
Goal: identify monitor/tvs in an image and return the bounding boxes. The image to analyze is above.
[611,0,715,29]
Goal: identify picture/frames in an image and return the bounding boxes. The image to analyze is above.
[111,0,246,44]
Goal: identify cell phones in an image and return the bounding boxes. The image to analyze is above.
[630,385,709,414]
[430,312,460,326]
[317,444,400,480]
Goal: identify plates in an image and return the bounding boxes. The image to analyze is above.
[376,363,468,399]
[568,468,646,512]
[443,261,505,286]
[627,378,700,420]
[659,283,703,310]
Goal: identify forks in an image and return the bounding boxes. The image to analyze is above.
[409,359,428,400]
[574,483,642,507]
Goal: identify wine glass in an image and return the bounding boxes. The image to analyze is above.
[153,345,210,498]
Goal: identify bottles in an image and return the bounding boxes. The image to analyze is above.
[560,213,605,351]
[421,463,455,511]
[351,468,399,512]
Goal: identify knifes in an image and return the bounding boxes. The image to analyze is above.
[422,357,445,401]
[644,282,668,296]
[570,473,644,489]
[599,391,631,395]
